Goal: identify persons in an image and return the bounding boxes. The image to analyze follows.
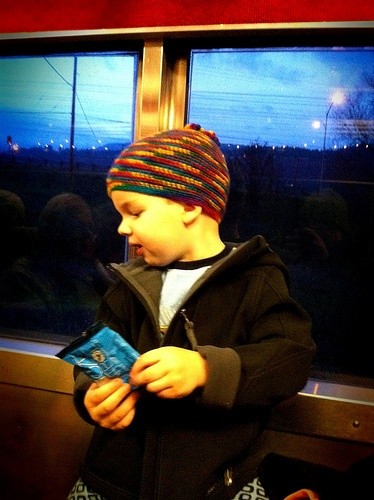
[43,123,321,500]
[273,184,356,372]
[26,193,116,336]
[0,190,61,334]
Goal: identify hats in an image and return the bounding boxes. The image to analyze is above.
[105,122,232,220]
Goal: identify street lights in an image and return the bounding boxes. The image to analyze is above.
[312,89,347,195]
